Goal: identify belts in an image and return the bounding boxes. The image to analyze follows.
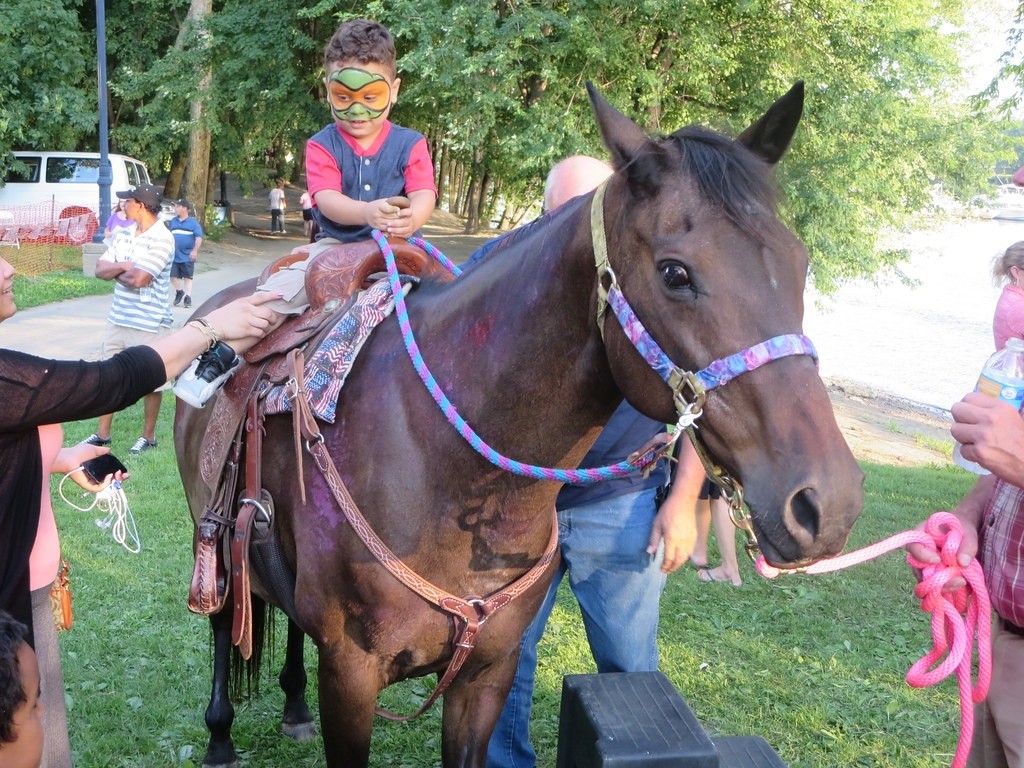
[995,617,1024,636]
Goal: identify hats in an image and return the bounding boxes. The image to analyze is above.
[116,183,163,208]
[172,199,190,209]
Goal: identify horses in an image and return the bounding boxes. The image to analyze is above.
[170,77,868,766]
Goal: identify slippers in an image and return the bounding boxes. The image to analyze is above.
[696,568,715,584]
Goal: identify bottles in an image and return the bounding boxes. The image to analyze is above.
[953,337,1024,476]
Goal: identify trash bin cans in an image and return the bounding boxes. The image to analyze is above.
[213,200,226,225]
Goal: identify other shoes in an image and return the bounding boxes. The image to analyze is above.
[302,233,308,237]
[280,231,286,235]
[271,231,277,235]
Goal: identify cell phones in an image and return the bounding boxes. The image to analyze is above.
[79,453,126,484]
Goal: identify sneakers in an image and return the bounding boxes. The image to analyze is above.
[173,341,242,409]
[82,434,112,447]
[130,438,158,455]
[184,295,191,308]
[173,289,185,306]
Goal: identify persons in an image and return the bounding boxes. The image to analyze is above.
[167,199,203,308]
[104,198,138,238]
[29,421,132,767]
[951,391,1024,768]
[77,183,175,455]
[485,155,706,768]
[0,258,284,647]
[0,612,45,767]
[299,185,313,236]
[690,476,741,586]
[989,241,1024,352]
[172,18,438,408]
[269,177,287,233]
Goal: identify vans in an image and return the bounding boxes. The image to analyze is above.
[1,149,153,246]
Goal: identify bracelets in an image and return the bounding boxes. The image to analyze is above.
[187,317,218,352]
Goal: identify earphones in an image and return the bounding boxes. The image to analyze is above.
[95,518,107,527]
[104,517,113,528]
